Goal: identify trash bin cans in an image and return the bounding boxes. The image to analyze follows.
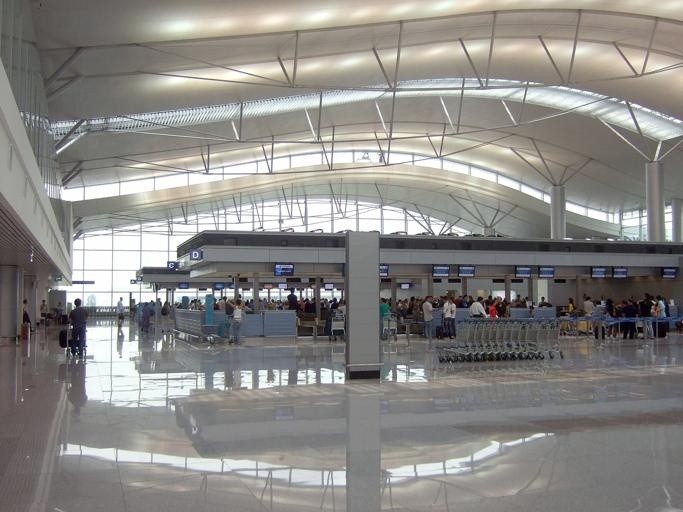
[21,323,30,341]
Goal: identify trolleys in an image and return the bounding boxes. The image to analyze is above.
[560,313,609,337]
[67,322,87,358]
[201,316,235,345]
[382,312,399,341]
[436,318,565,363]
[329,315,347,342]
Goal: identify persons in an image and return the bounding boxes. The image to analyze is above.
[116,296,125,328]
[23,298,35,332]
[69,299,88,357]
[55,301,65,325]
[39,300,48,323]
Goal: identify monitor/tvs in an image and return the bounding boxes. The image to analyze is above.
[379,265,389,277]
[432,266,450,277]
[179,282,410,290]
[591,268,606,278]
[515,267,531,278]
[612,268,627,279]
[663,269,677,279]
[538,267,554,278]
[274,264,294,276]
[458,266,475,277]
